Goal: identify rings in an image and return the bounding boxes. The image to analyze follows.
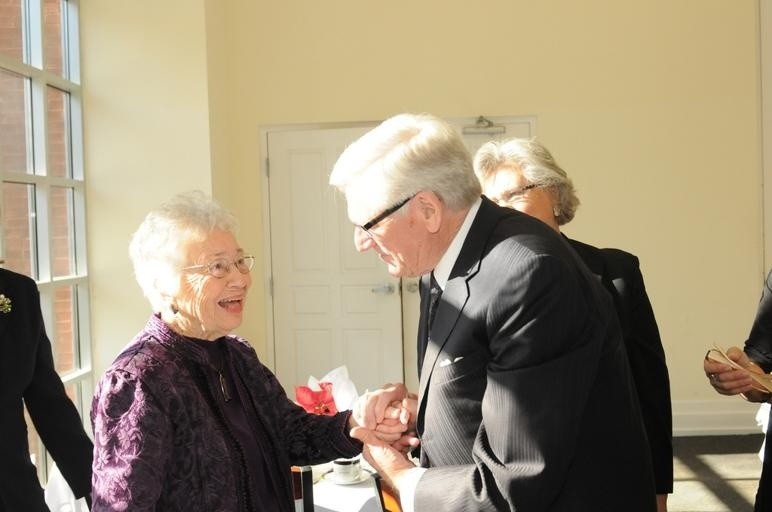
[708,372,717,379]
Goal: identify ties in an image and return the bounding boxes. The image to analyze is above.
[426,276,444,343]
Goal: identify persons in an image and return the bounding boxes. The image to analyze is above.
[472,133,675,511]
[700,263,772,510]
[1,267,97,512]
[327,112,661,511]
[89,188,420,512]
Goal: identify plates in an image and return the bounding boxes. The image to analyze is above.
[322,469,373,485]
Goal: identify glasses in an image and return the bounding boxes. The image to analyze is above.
[491,181,543,206]
[180,255,257,280]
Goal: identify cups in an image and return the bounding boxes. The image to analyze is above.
[333,455,361,482]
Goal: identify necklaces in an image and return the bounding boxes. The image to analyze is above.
[208,355,233,403]
